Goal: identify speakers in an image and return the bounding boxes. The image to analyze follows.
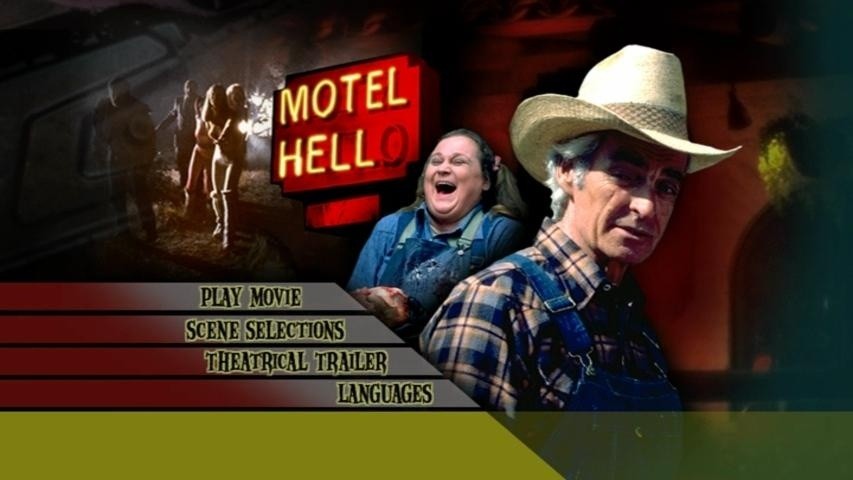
[218,189,235,254]
[208,190,223,239]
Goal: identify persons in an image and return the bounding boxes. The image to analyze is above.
[153,80,203,195]
[418,45,740,480]
[93,76,160,254]
[347,128,524,345]
[182,82,253,216]
[203,85,244,248]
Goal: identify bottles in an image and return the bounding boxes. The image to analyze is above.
[508,45,744,185]
